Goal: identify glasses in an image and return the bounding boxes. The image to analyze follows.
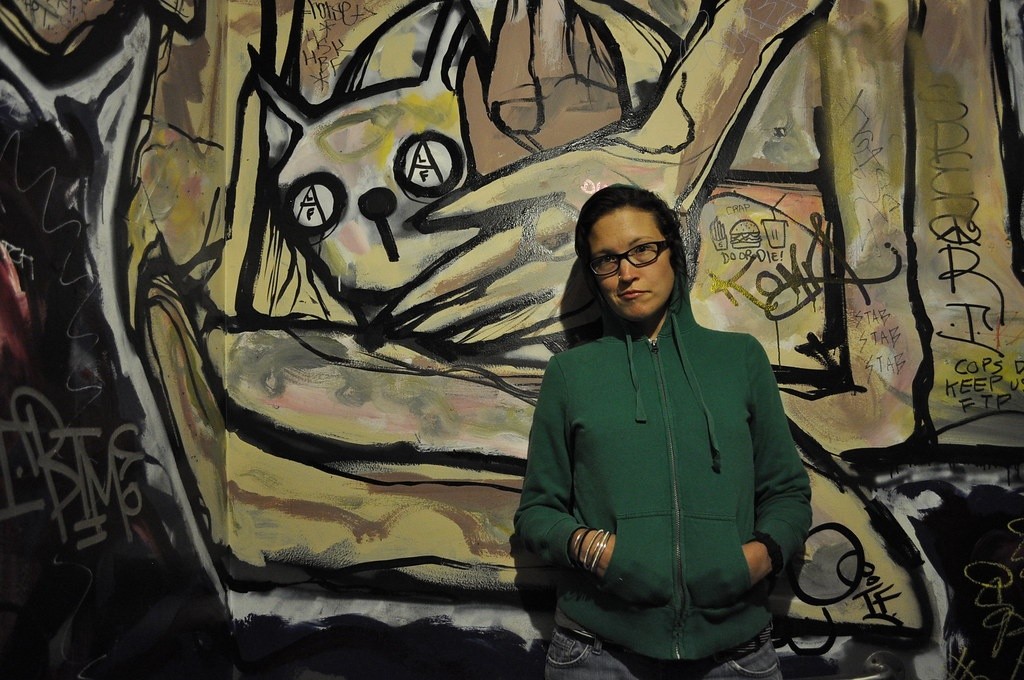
[587,241,673,275]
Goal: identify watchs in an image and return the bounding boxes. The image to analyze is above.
[752,528,783,574]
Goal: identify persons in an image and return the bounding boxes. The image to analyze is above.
[512,183,813,680]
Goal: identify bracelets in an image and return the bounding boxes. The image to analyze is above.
[572,528,590,560]
[590,530,611,574]
[583,529,605,570]
[576,528,598,564]
